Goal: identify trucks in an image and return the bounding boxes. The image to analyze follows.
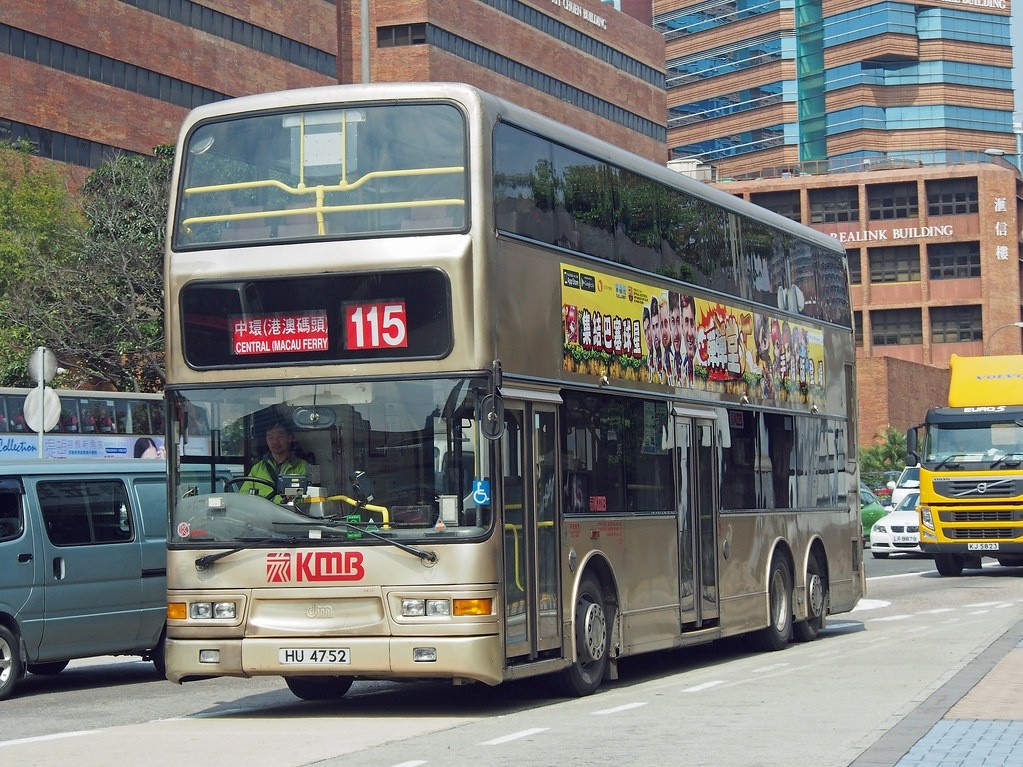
[906,353,1023,579]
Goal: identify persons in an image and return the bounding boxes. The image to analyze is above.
[658,292,670,348]
[237,416,311,505]
[667,291,683,353]
[133,438,165,459]
[679,294,697,357]
[650,296,660,349]
[642,307,652,350]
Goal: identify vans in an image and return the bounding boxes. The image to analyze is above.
[887,463,920,509]
[0,459,240,700]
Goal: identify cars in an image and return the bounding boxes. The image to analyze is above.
[869,493,925,560]
[860,470,902,547]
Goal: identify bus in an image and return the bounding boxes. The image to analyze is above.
[163,82,867,699]
[0,387,212,459]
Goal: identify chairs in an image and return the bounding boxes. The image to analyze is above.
[218,199,328,242]
[0,411,117,434]
[400,197,456,229]
